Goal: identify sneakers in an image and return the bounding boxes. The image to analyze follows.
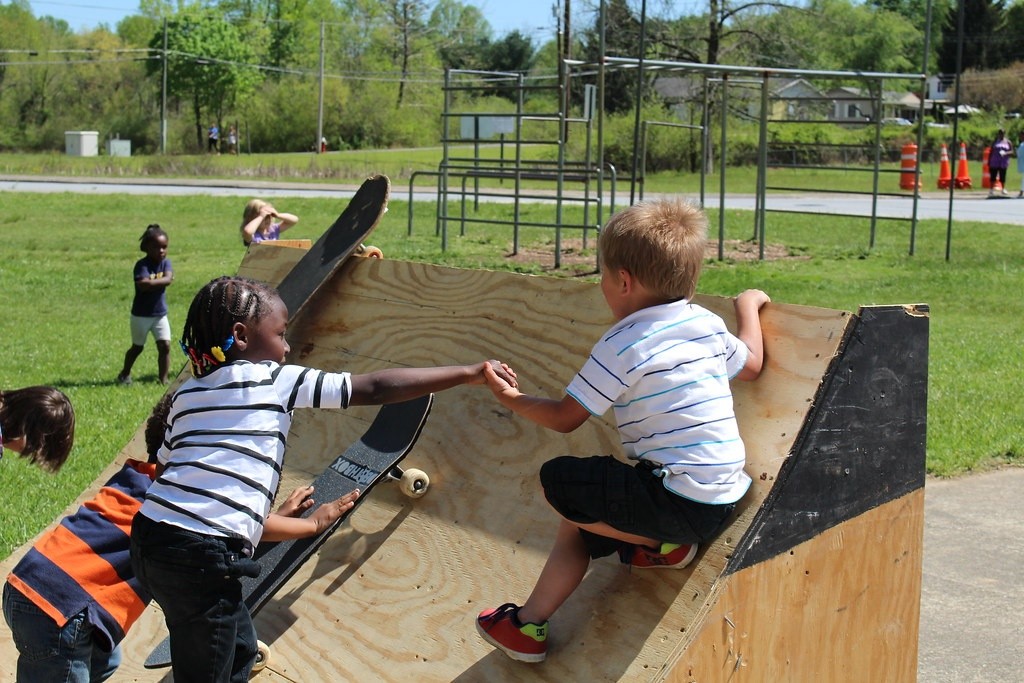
[475,603,549,663]
[629,542,699,569]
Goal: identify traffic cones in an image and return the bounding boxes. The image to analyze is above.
[937,143,955,189]
[954,143,972,190]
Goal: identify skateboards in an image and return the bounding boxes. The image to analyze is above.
[265,169,395,333]
[141,386,443,672]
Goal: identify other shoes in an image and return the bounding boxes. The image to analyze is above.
[988,188,1009,196]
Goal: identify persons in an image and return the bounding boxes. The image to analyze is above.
[240,197,299,247]
[1015,130,1024,198]
[1,392,362,683]
[117,223,173,387]
[227,124,238,155]
[988,128,1014,197]
[0,384,76,475]
[130,274,519,683]
[476,197,772,664]
[206,123,222,156]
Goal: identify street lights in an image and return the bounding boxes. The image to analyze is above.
[536,25,563,141]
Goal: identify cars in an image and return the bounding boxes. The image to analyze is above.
[880,118,909,126]
